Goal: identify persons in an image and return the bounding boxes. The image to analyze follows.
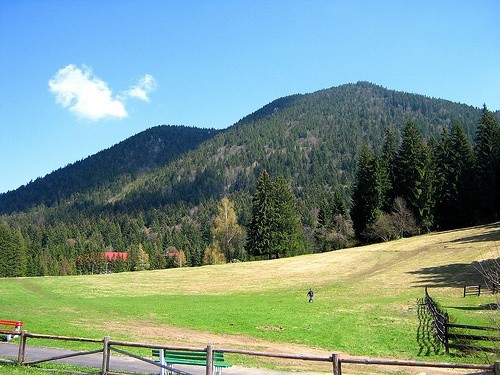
[307,288,314,303]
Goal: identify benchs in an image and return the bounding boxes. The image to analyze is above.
[151,349,232,375]
[0,320,24,342]
